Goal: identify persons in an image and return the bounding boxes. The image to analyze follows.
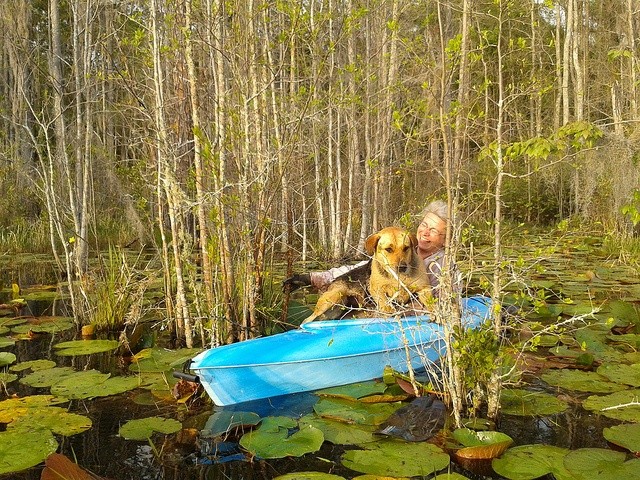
[282,199,463,311]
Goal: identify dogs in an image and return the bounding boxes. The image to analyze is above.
[300,227,446,329]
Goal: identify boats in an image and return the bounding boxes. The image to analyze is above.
[190,295,493,408]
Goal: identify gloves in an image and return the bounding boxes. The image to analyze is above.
[281,274,310,295]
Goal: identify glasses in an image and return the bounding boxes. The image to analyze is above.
[419,221,447,236]
